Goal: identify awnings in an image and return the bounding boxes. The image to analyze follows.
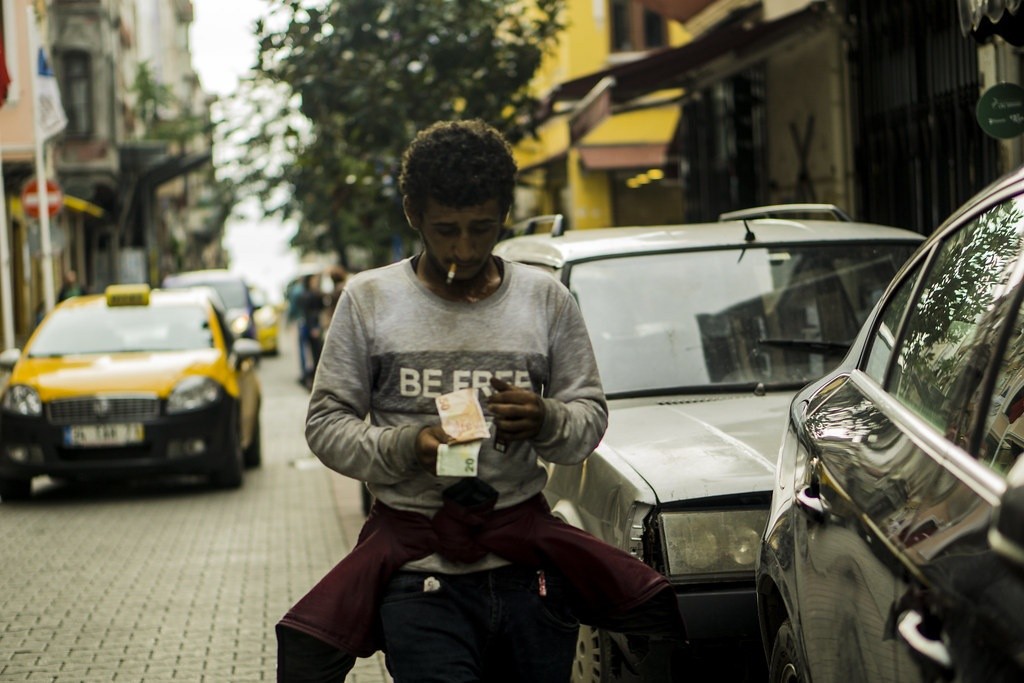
[957,0,1024,48]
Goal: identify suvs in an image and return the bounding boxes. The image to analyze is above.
[482,200,933,683]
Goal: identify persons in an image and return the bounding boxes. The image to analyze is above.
[286,121,608,682]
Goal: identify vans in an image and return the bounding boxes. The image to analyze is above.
[159,272,265,361]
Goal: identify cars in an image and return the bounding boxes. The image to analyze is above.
[764,171,1023,683]
[248,283,282,354]
[0,283,267,495]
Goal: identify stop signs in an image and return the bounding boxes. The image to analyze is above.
[23,177,61,218]
